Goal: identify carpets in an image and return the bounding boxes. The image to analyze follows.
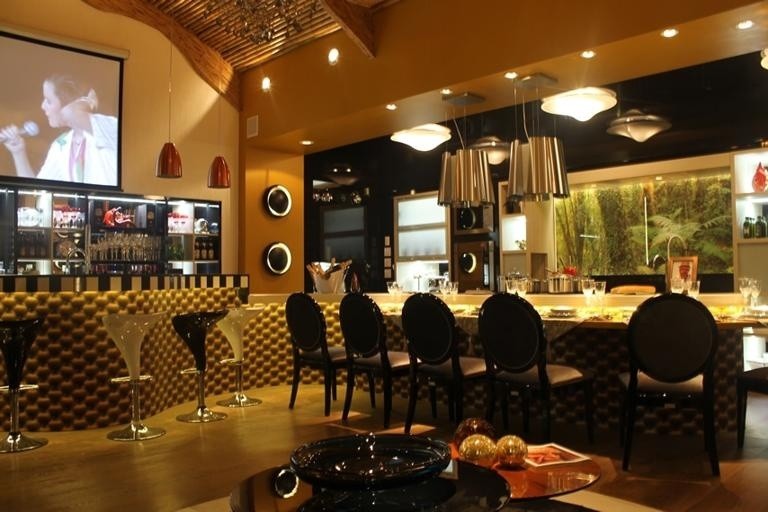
[175,477,663,511]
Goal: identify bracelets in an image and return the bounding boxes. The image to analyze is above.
[80,96,97,112]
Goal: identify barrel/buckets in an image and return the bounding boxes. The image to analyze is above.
[439,280,459,301]
[387,282,404,302]
[582,281,606,305]
[311,261,349,294]
[506,278,526,298]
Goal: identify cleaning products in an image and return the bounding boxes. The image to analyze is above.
[743,215,768,238]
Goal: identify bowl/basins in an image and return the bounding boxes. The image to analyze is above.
[289,431,451,491]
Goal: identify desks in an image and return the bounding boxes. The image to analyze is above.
[228,457,510,512]
[454,450,602,511]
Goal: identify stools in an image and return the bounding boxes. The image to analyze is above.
[0,316,49,453]
[216,306,265,406]
[171,310,228,423]
[102,311,166,441]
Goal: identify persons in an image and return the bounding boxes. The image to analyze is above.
[0,70,118,187]
[673,261,693,289]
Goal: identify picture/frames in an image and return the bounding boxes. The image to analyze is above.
[666,256,700,294]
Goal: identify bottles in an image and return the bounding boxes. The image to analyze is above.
[194,240,215,260]
[743,215,767,238]
[17,233,47,258]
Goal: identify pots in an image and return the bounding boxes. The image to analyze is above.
[544,275,578,294]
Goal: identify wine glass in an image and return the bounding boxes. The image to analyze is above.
[52,209,84,229]
[167,217,191,234]
[438,279,459,310]
[387,280,403,308]
[738,277,762,312]
[581,279,607,314]
[90,234,162,261]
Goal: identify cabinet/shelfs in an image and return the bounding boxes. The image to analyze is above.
[0,177,222,277]
[394,188,450,292]
[498,182,547,293]
[730,148,767,294]
[318,200,372,293]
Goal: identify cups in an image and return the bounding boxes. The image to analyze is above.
[504,278,528,298]
[669,278,701,299]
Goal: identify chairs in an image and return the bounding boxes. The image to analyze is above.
[339,293,437,428]
[618,293,720,476]
[284,293,376,415]
[401,293,503,433]
[479,292,594,446]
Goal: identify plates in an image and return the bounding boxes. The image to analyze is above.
[551,305,577,313]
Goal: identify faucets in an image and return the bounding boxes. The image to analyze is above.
[667,235,688,293]
[65,249,89,269]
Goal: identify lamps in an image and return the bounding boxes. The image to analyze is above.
[154,1,184,178]
[207,39,231,188]
[199,0,318,43]
[436,91,495,210]
[505,75,569,204]
[391,84,673,167]
